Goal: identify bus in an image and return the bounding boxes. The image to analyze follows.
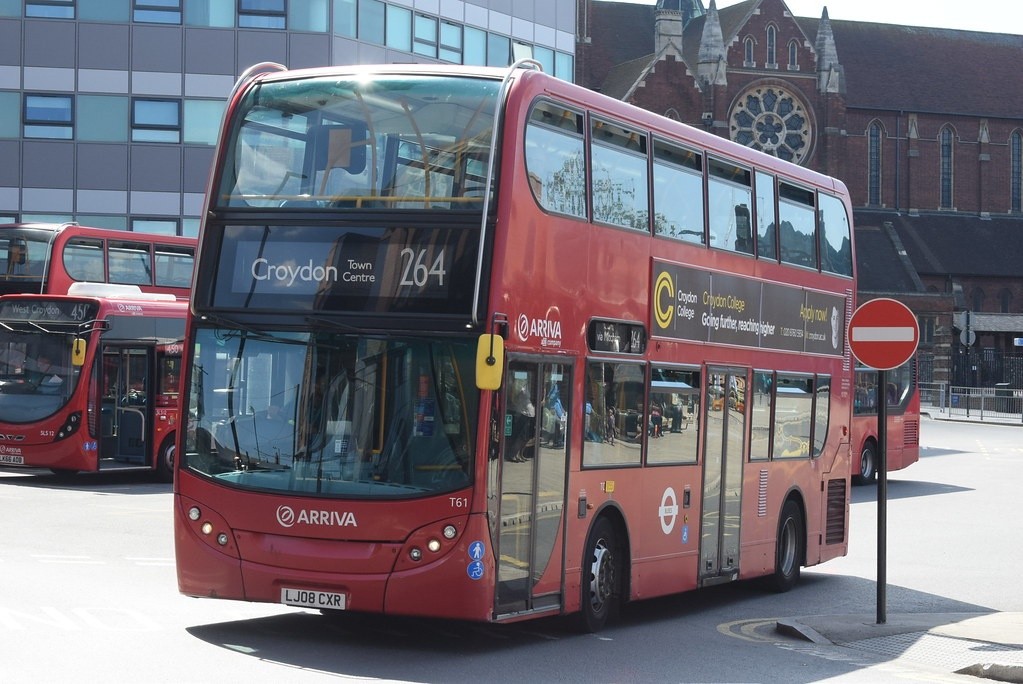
[153,57,861,636]
[848,306,922,487]
[0,221,200,486]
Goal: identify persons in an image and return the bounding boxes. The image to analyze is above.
[766,378,772,406]
[650,400,664,438]
[18,353,51,383]
[831,306,839,349]
[267,364,331,432]
[671,392,682,433]
[509,364,547,463]
[607,407,616,446]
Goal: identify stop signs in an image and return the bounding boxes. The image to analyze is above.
[847,296,921,371]
